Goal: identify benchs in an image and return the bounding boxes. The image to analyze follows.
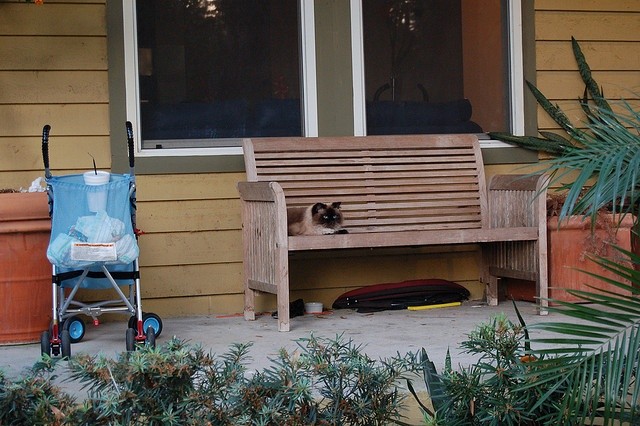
[237,134,552,331]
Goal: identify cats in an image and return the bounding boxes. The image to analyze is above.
[286,199,349,237]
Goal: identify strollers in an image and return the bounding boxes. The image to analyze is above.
[41,120,163,360]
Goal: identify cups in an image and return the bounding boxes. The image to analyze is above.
[82,172,111,212]
